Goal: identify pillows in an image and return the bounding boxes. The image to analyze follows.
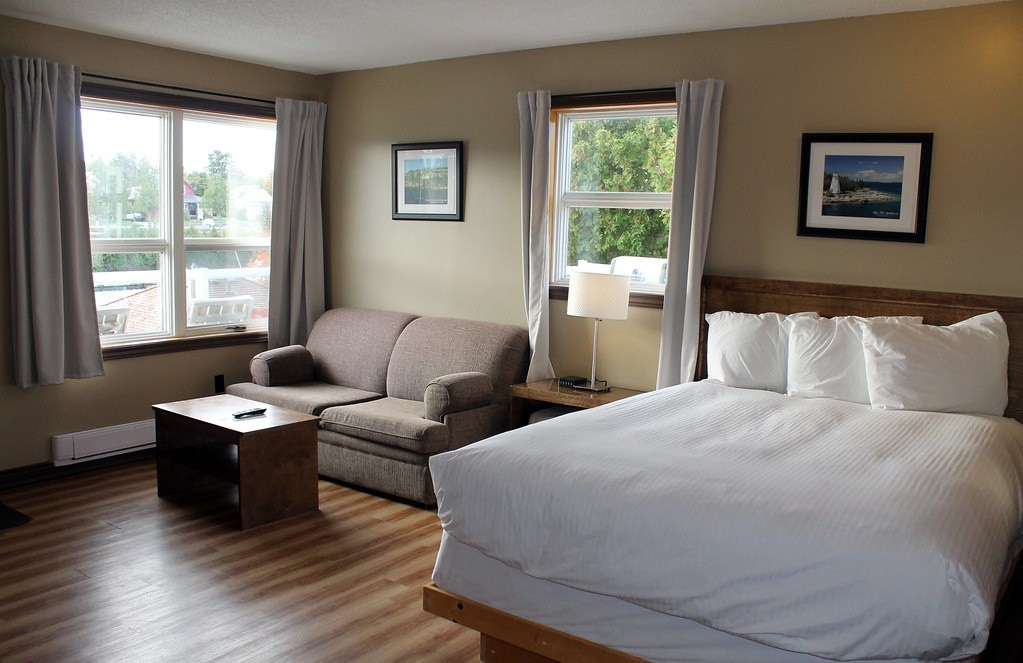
[785,315,923,404]
[854,311,1011,417]
[704,311,819,394]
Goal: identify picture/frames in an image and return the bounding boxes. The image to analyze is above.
[391,140,463,222]
[796,132,935,244]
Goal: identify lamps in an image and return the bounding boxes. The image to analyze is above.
[567,272,630,392]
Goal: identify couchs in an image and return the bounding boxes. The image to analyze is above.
[224,308,530,507]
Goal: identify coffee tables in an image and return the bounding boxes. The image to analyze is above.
[152,394,323,530]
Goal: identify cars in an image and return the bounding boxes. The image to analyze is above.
[123,213,134,222]
[133,213,145,222]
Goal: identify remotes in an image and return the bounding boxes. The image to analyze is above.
[232,408,266,418]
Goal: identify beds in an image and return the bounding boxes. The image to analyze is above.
[422,277,1023,663]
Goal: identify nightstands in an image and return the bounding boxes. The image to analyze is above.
[508,377,644,433]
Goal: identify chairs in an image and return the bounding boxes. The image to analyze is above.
[97,305,132,336]
[188,296,255,324]
